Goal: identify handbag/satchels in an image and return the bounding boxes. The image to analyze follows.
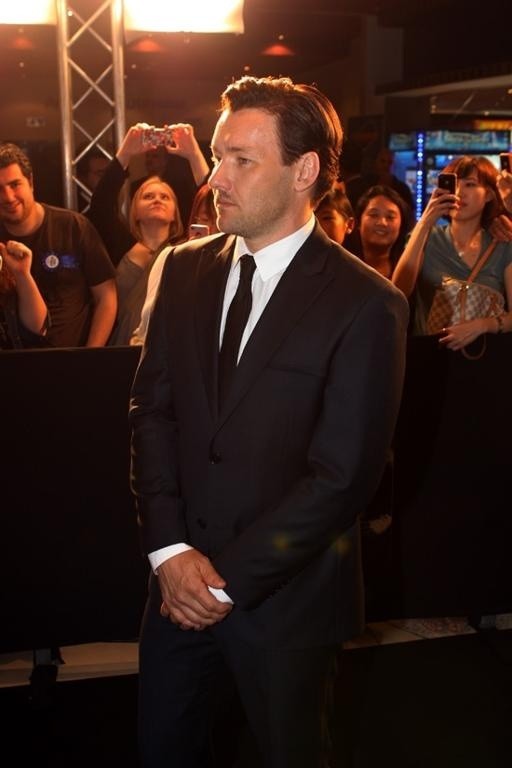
[426,277,505,334]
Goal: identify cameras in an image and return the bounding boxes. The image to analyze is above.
[143,128,174,146]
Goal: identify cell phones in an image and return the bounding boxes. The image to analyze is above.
[189,224,210,238]
[500,153,512,173]
[438,174,455,209]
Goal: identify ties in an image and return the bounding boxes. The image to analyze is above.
[216,254,256,408]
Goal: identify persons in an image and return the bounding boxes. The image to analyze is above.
[1,123,222,351]
[314,146,512,351]
[127,75,411,768]
